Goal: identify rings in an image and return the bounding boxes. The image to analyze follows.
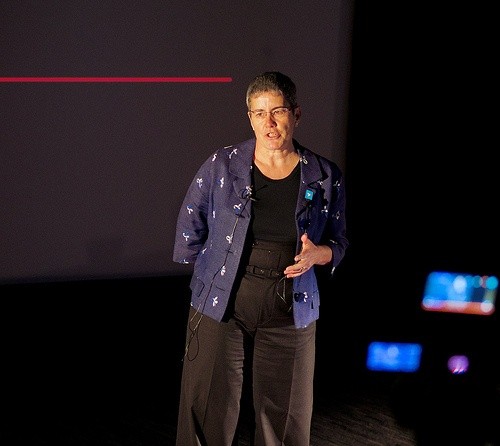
[301,266,306,272]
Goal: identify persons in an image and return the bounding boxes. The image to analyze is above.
[172,72,349,446]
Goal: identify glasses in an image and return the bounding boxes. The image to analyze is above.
[248,102,296,120]
